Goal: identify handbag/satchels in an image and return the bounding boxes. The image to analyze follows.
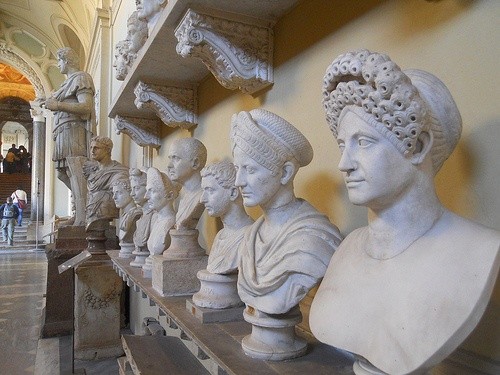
[18,199,28,209]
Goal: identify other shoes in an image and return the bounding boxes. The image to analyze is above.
[3,238,8,241]
[18,225,22,227]
[8,244,14,246]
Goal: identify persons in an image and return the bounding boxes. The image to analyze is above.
[37,45,97,226]
[229,106,343,315]
[83,135,126,232]
[133,0,168,35]
[0,196,21,246]
[167,135,208,231]
[308,48,500,374]
[4,143,32,174]
[112,179,143,242]
[128,166,154,248]
[112,40,138,82]
[11,184,28,227]
[144,166,178,257]
[125,10,150,55]
[198,157,254,275]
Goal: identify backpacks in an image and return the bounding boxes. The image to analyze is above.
[2,203,15,217]
